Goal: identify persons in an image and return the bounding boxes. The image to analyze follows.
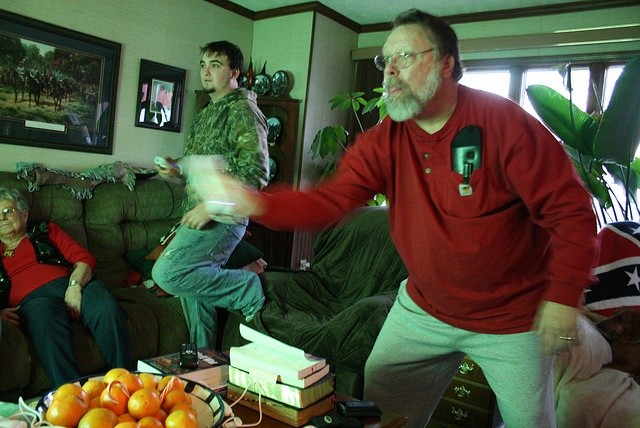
[15,54,77,89]
[151,39,270,350]
[0,186,128,390]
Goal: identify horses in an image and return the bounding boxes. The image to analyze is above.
[10,69,25,103]
[48,77,67,112]
[24,74,41,108]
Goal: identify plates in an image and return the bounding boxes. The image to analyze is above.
[33,370,226,427]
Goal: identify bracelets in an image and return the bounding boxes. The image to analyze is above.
[66,279,82,289]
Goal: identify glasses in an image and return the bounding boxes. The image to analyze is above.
[370,46,438,70]
[0,204,23,216]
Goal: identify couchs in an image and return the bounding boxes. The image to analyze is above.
[1,161,198,399]
[224,206,409,394]
[553,221,636,426]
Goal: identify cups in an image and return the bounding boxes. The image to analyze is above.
[179,342,198,368]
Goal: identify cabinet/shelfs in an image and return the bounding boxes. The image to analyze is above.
[427,356,502,426]
[193,89,302,267]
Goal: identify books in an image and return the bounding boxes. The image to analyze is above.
[227,324,337,428]
[136,347,230,388]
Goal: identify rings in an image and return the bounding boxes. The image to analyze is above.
[74,310,77,313]
[558,333,577,341]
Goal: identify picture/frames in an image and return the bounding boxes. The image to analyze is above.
[135,58,186,132]
[0,8,122,155]
